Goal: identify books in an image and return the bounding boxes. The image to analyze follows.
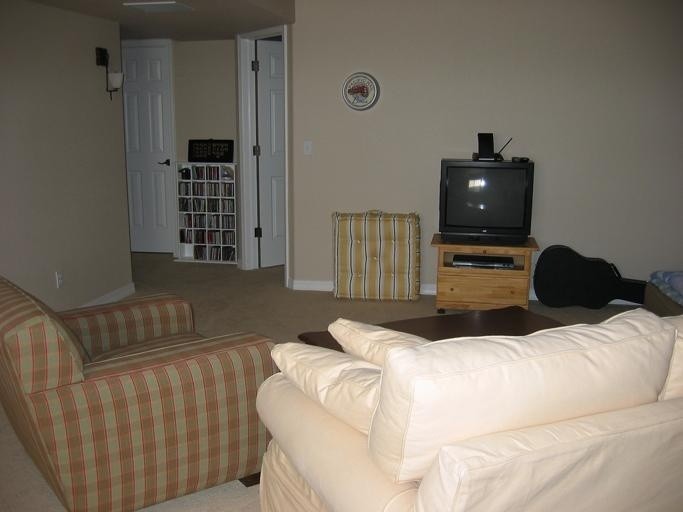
[178,166,235,262]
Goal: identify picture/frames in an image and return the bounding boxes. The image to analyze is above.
[341,71,380,110]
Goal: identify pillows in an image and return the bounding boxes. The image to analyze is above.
[326,317,432,365]
[269,341,382,438]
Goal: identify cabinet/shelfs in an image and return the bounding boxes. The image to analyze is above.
[428,233,539,315]
[172,162,239,264]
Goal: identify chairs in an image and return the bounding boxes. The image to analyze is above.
[0,271,280,512]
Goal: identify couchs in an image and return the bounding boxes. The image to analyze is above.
[254,305,682,511]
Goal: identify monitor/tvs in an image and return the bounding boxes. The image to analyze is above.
[439,158,535,235]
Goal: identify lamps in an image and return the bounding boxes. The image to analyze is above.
[95,47,122,102]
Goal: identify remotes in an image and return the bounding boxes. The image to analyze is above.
[520,158,529,162]
[512,157,520,162]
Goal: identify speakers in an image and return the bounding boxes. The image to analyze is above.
[478,133,494,157]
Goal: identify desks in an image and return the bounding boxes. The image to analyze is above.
[299,304,565,354]
